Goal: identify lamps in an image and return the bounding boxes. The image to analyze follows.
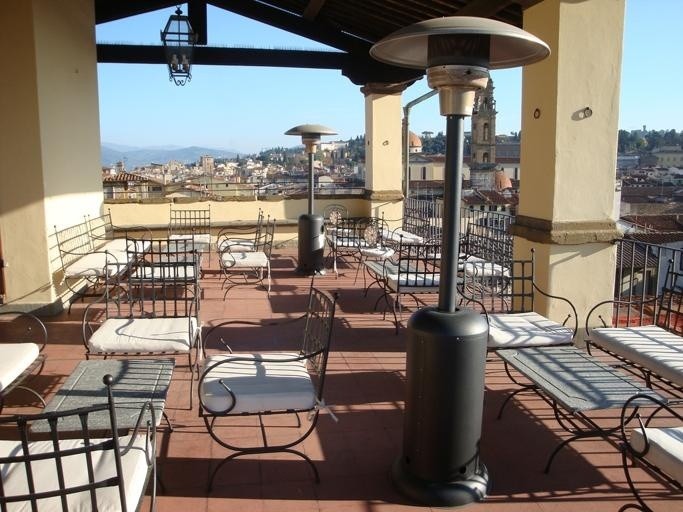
[159,4,197,86]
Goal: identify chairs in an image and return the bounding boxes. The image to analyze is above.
[201,268,341,494]
[84,225,206,412]
[458,249,578,383]
[616,394,681,511]
[0,374,159,511]
[51,205,517,317]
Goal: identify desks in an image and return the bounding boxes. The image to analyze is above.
[28,358,175,434]
[494,347,669,475]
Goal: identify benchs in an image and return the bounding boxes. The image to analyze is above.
[1,310,50,412]
[584,258,682,392]
